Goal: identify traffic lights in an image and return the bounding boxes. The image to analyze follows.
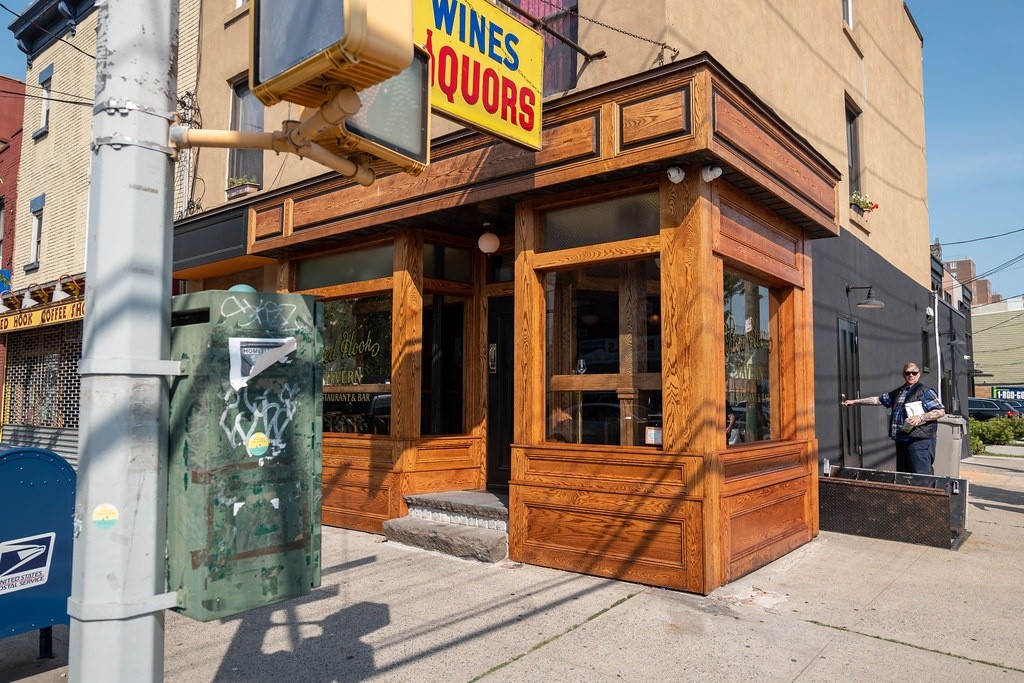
[249,0,350,92]
[336,43,432,176]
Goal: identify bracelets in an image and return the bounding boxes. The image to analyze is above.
[919,414,925,421]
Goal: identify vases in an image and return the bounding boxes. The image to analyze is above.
[850,202,865,217]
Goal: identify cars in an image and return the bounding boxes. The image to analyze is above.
[552,405,661,446]
[367,394,391,433]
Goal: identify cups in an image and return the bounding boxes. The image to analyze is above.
[903,418,914,433]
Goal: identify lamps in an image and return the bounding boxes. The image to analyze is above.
[0,290,21,313]
[21,283,51,310]
[51,273,83,304]
[846,284,886,309]
[478,223,500,257]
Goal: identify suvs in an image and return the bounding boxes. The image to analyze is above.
[965,397,1020,421]
[991,398,1024,418]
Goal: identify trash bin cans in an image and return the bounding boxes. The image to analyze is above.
[933,414,967,478]
[0,444,75,642]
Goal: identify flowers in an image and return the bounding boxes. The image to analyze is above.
[849,190,878,213]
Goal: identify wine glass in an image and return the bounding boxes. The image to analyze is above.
[577,359,586,374]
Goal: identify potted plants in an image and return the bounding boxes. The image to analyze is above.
[224,175,260,201]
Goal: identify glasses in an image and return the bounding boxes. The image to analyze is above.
[903,370,920,376]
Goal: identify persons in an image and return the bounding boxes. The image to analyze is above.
[726,401,737,444]
[846,362,946,475]
